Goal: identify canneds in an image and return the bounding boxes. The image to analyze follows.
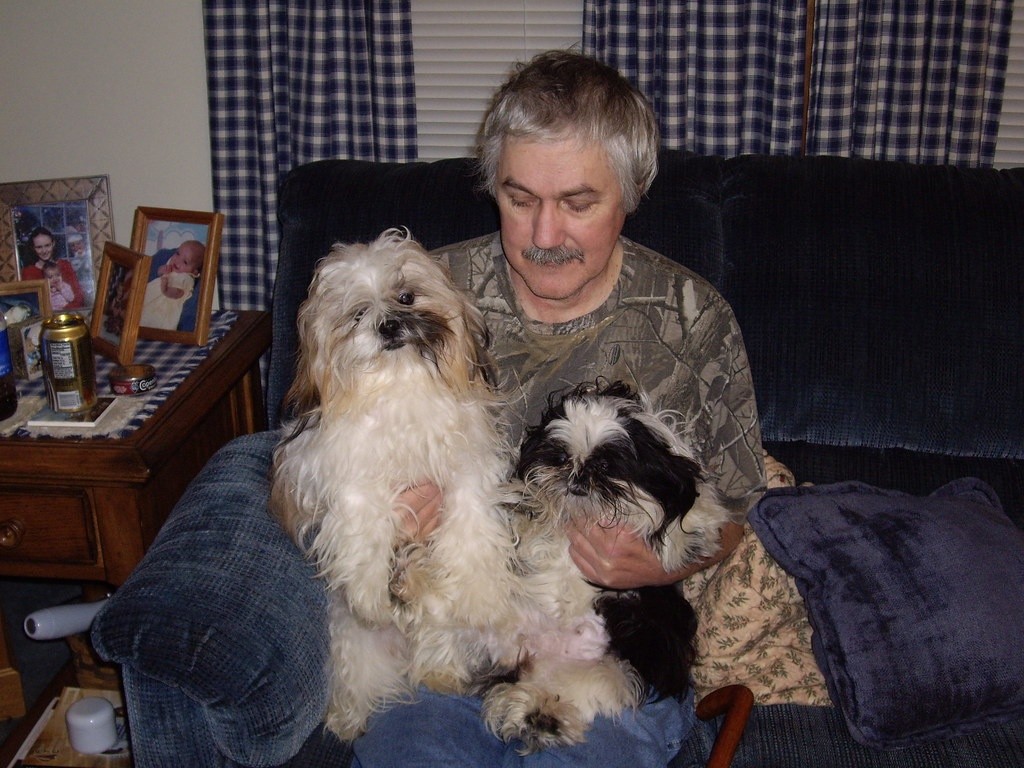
[38,313,98,414]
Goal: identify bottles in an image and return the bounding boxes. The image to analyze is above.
[0,311,18,422]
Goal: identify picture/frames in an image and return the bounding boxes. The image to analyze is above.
[89,241,152,367]
[130,206,225,347]
[0,173,115,321]
[0,278,53,319]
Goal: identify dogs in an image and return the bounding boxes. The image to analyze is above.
[271,224,644,756]
[506,385,728,698]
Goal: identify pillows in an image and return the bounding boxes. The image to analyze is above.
[740,475,1024,750]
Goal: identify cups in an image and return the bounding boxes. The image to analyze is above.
[66,697,118,755]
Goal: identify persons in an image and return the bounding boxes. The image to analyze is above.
[137,239,205,332]
[267,50,768,767]
[19,226,94,312]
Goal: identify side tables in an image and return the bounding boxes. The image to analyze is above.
[0,307,270,768]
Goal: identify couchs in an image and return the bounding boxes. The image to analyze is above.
[90,150,1024,768]
